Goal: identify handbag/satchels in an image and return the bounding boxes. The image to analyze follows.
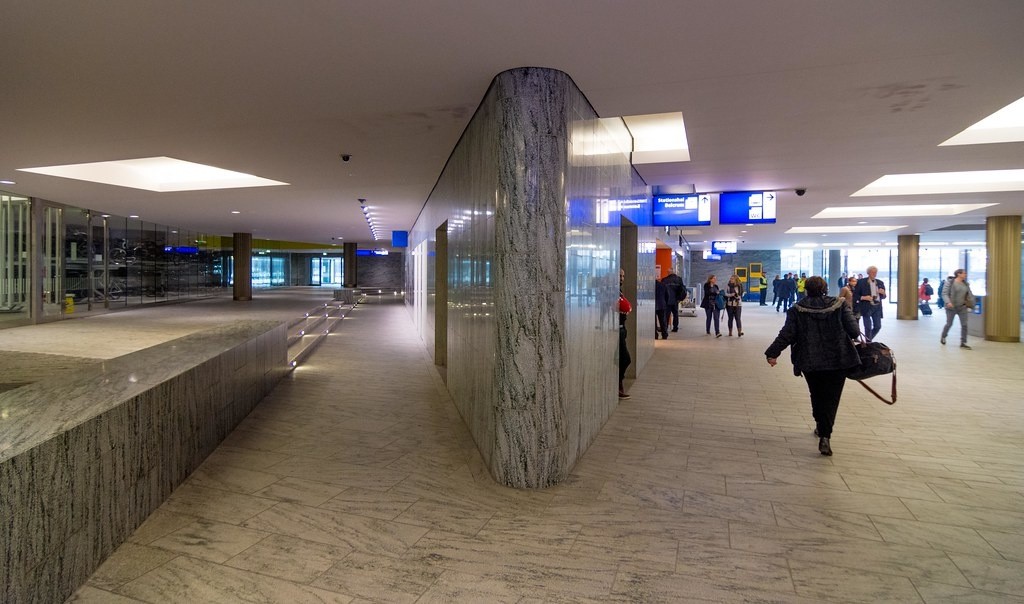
[845,331,897,381]
[716,289,727,310]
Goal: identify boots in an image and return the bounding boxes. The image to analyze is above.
[729,329,732,336]
[738,327,744,336]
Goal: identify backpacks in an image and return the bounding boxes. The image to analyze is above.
[924,283,933,295]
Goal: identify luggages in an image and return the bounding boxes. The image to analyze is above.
[920,301,932,315]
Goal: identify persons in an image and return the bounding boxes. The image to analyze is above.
[724,275,744,336]
[839,277,861,343]
[918,277,931,305]
[618,269,632,399]
[758,272,768,306]
[940,269,976,351]
[855,266,887,342]
[838,272,848,288]
[659,268,687,332]
[700,274,723,338]
[857,273,864,281]
[938,279,946,307]
[655,275,669,340]
[765,277,861,456]
[772,273,808,313]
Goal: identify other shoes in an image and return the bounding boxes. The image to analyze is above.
[941,336,946,344]
[672,327,677,331]
[819,435,833,455]
[662,335,666,339]
[619,380,630,399]
[814,424,819,436]
[716,333,721,337]
[961,343,971,349]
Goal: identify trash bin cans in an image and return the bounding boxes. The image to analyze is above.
[966,295,985,338]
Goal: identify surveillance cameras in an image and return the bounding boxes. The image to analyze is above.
[341,153,352,162]
[795,188,807,196]
